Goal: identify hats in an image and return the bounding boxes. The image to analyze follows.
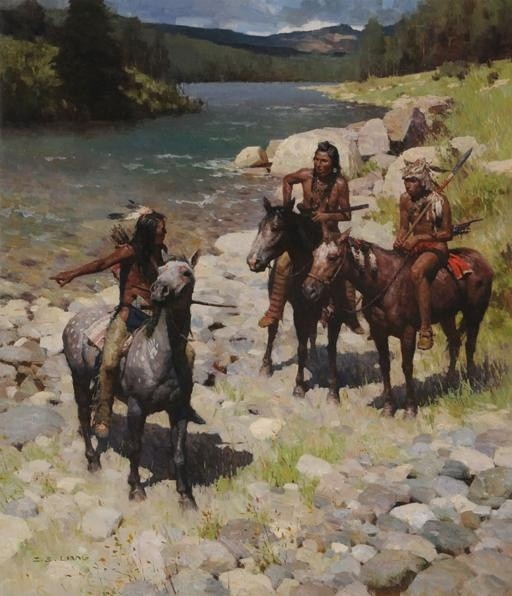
[403,164,432,192]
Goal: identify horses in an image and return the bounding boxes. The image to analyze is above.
[62,247,202,512]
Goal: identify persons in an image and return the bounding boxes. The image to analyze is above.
[40,208,206,443]
[368,155,453,350]
[254,138,368,337]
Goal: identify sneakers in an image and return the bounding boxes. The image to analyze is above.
[259,315,274,328]
[190,409,205,425]
[94,420,107,439]
[418,327,433,349]
[351,319,363,334]
